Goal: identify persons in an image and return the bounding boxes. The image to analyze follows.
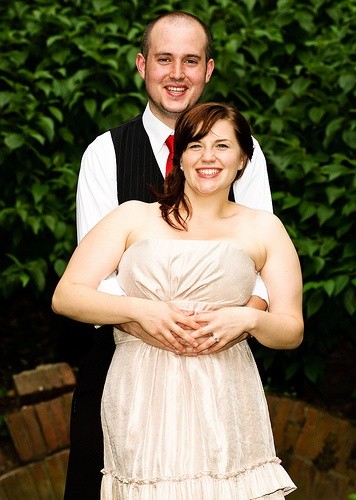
[63,10,274,500]
[50,104,304,500]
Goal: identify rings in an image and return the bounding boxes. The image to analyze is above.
[211,334,220,342]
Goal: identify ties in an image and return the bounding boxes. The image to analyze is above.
[165,135,174,177]
